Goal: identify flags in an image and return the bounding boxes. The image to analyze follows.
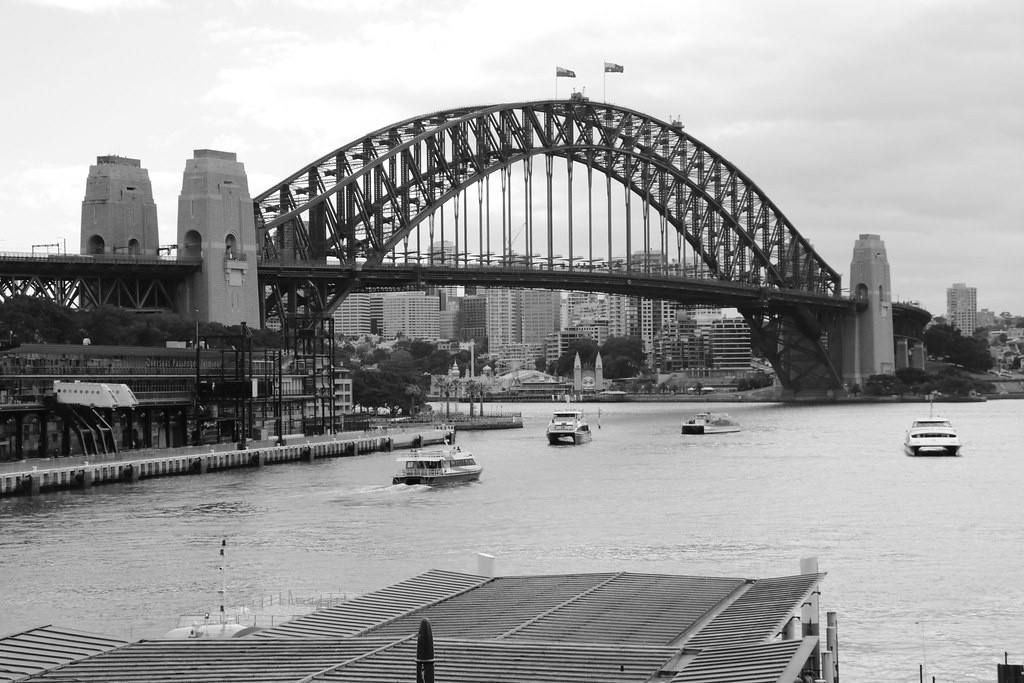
[604,61,624,73]
[556,66,576,78]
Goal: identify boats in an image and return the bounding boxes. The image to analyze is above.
[598,391,627,402]
[682,402,742,435]
[545,408,592,445]
[392,439,482,486]
[904,400,962,457]
